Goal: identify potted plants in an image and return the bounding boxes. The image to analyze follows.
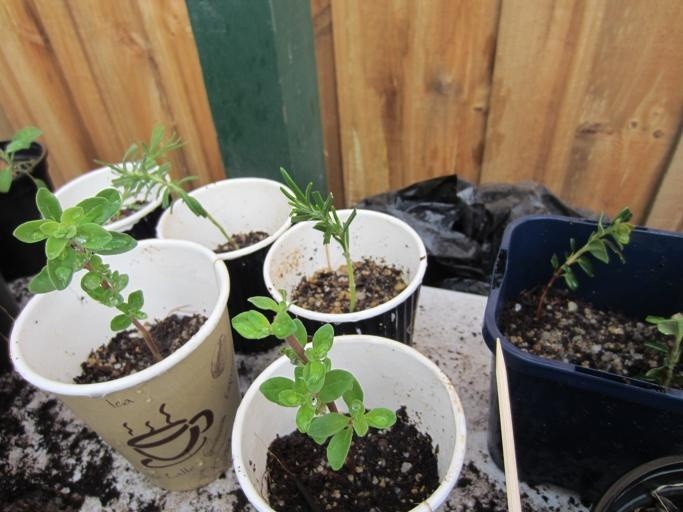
[0,121,57,283]
[481,205,682,492]
[94,124,300,354]
[6,190,242,493]
[231,291,469,509]
[41,160,173,244]
[263,165,428,353]
